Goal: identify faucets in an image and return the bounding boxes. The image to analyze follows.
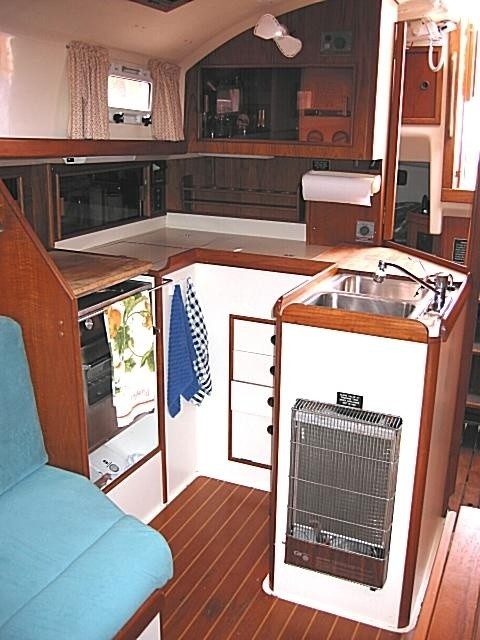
[374,259,449,313]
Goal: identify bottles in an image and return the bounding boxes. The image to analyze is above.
[203,74,267,140]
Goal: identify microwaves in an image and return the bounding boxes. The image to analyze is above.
[57,160,169,242]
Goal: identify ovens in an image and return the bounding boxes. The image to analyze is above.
[74,281,155,456]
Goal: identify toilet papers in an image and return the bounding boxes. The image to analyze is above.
[301,172,381,208]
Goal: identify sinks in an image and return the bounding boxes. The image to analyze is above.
[303,291,417,318]
[334,274,432,304]
